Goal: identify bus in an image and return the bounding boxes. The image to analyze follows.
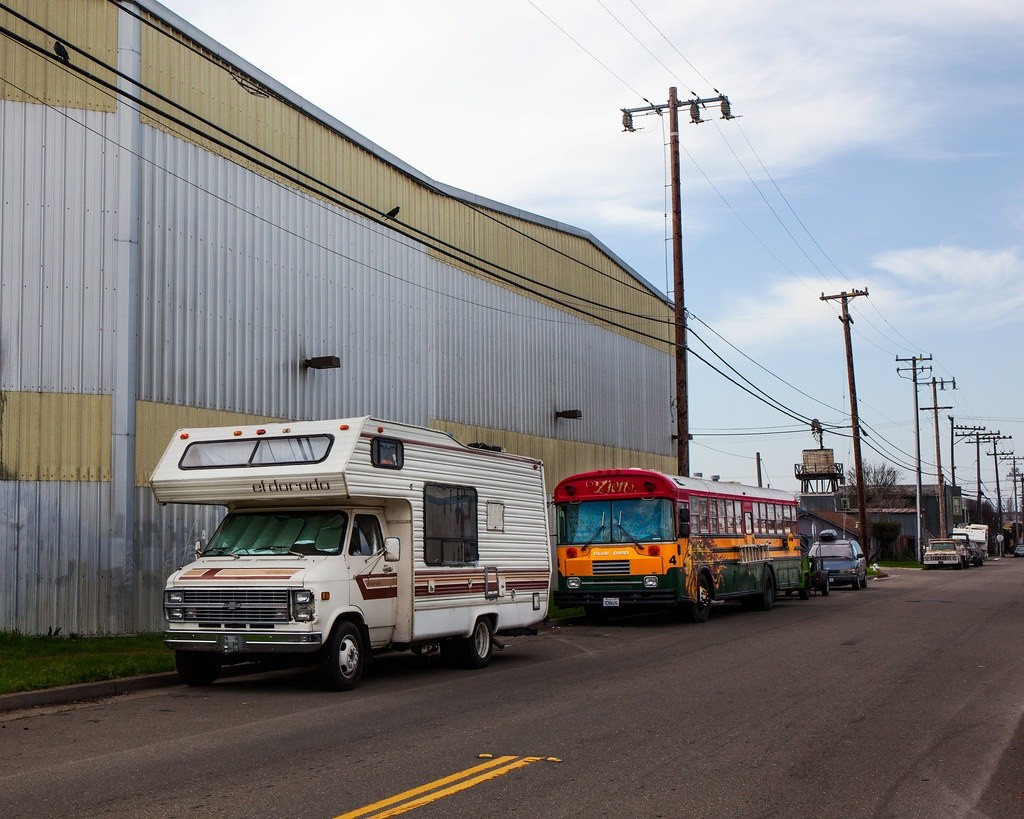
[547,467,802,623]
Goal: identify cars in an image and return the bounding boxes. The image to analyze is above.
[968,541,983,567]
[1014,546,1024,557]
[923,540,964,569]
[955,540,970,567]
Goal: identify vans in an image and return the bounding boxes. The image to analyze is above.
[146,415,553,689]
[808,529,868,590]
